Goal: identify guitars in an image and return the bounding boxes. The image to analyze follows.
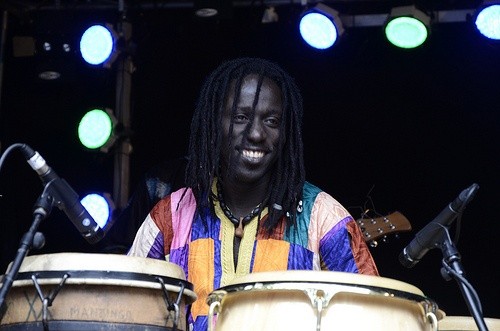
[355,209,413,247]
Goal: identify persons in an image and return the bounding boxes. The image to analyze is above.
[122,54,386,331]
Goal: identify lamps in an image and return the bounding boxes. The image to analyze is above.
[76,108,122,152]
[28,50,69,80]
[381,0,436,52]
[297,2,346,50]
[78,22,123,70]
[470,0,500,46]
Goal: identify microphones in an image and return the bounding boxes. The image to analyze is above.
[399,183,478,269]
[19,144,104,244]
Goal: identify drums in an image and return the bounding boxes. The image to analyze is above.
[0,253,198,331]
[205,271,437,331]
[430,315,500,331]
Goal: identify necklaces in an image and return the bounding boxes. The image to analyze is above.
[215,175,275,225]
